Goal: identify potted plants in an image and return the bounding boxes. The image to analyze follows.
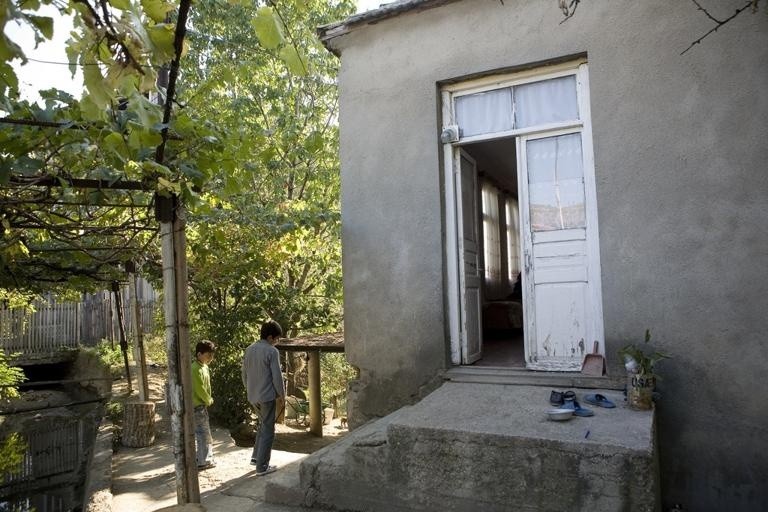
[619,329,672,410]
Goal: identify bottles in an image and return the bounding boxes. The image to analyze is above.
[623,354,640,372]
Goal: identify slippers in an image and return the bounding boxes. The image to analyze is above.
[559,401,593,417]
[583,390,616,409]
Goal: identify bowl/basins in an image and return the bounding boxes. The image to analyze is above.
[543,407,575,421]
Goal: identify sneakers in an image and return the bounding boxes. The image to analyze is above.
[250,459,276,476]
[551,391,575,405]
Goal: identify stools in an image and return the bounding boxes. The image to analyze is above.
[485,300,521,329]
[121,400,157,447]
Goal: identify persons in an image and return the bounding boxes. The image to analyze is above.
[191,339,218,470]
[241,319,286,476]
